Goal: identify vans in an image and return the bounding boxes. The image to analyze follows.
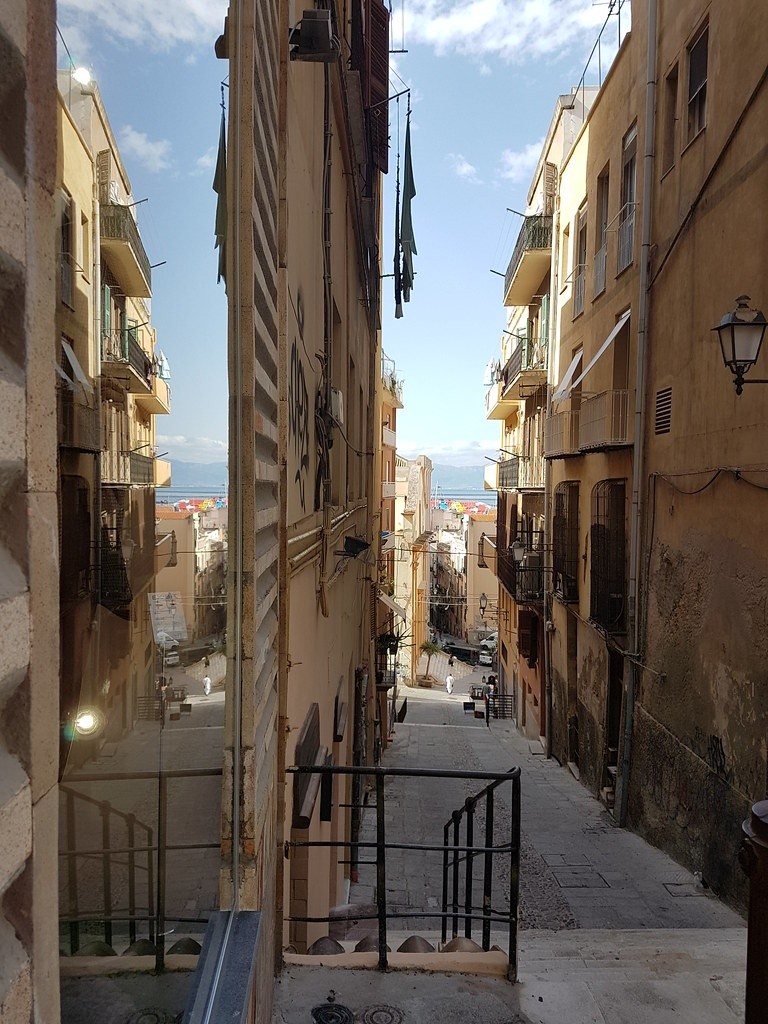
[480,631,498,649]
[440,644,480,666]
[158,632,181,651]
[178,645,212,666]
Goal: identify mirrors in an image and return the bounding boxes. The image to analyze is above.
[57,1,242,1022]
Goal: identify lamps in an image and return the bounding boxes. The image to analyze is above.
[478,593,499,614]
[710,295,768,396]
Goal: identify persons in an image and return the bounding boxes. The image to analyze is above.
[482,676,486,683]
[205,675,212,696]
[222,627,227,644]
[482,682,491,699]
[446,674,454,694]
[168,677,173,686]
[448,655,454,666]
[204,656,210,668]
[164,683,173,710]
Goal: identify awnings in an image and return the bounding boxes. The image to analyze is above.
[374,588,406,638]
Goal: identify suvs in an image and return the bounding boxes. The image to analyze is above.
[479,650,492,666]
[165,651,179,668]
